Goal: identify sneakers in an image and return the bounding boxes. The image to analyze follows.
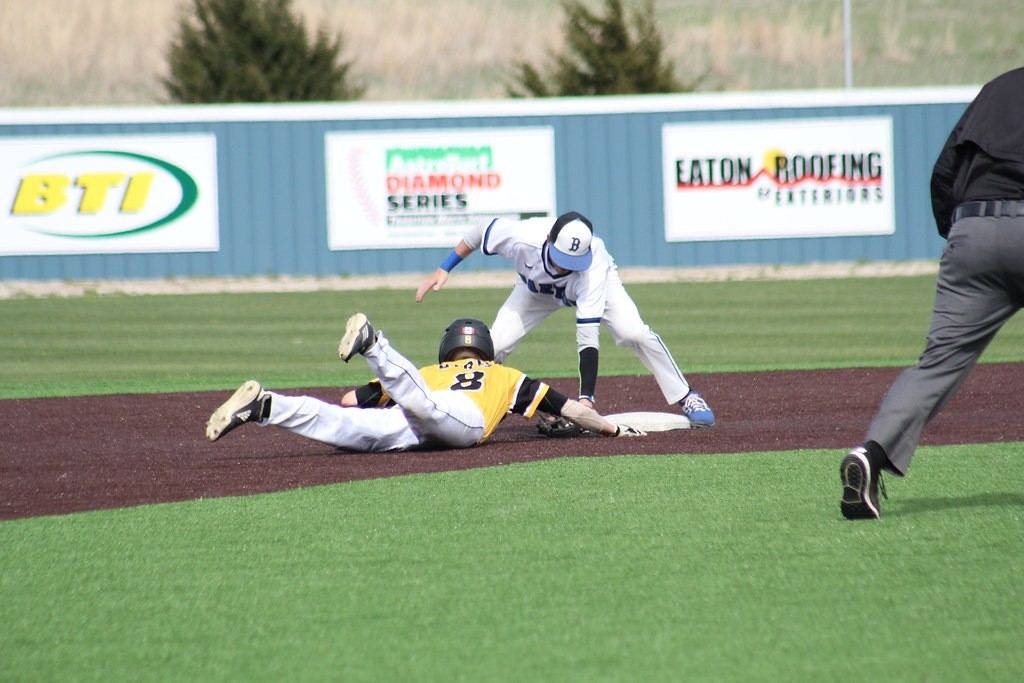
[839,445,888,520]
[338,313,374,362]
[679,389,715,426]
[206,380,270,442]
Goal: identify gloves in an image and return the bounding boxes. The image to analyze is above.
[611,423,647,437]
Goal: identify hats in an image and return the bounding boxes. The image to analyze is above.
[548,211,593,270]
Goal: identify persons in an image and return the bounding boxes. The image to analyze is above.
[205,312,647,451]
[415,212,717,426]
[839,66,1024,518]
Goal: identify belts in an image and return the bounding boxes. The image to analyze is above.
[954,202,1024,221]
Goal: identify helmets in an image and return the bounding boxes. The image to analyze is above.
[439,318,494,363]
